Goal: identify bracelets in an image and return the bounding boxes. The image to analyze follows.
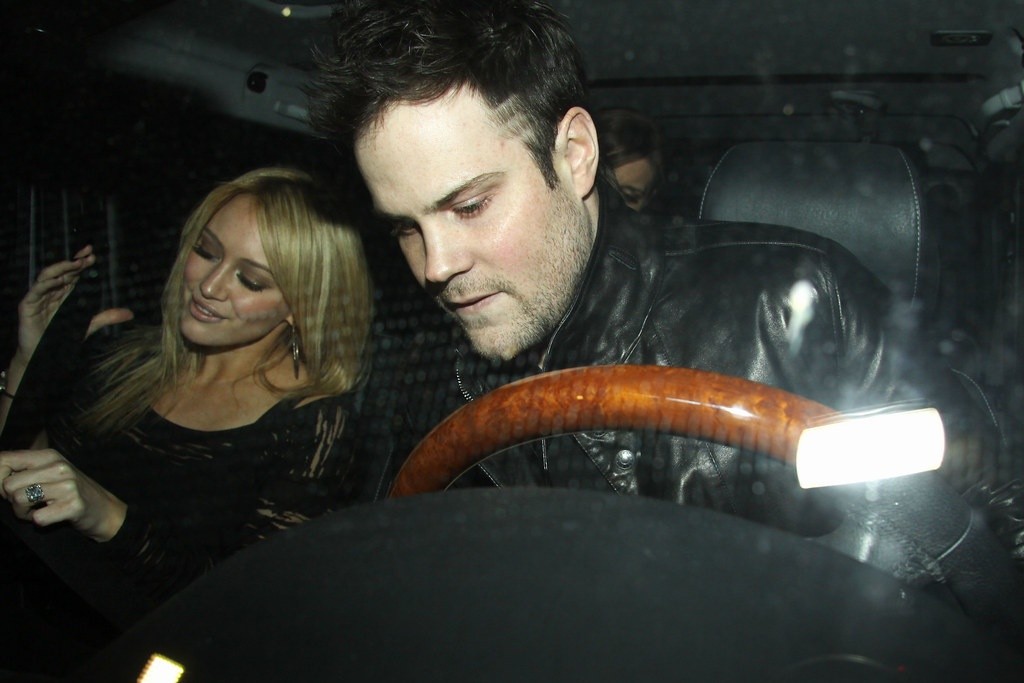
[1,388,14,400]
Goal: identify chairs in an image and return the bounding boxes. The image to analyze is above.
[695,137,934,328]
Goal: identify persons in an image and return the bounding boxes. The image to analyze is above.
[593,101,677,210]
[298,0,1024,571]
[0,166,373,683]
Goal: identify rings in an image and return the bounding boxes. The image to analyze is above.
[25,484,43,501]
